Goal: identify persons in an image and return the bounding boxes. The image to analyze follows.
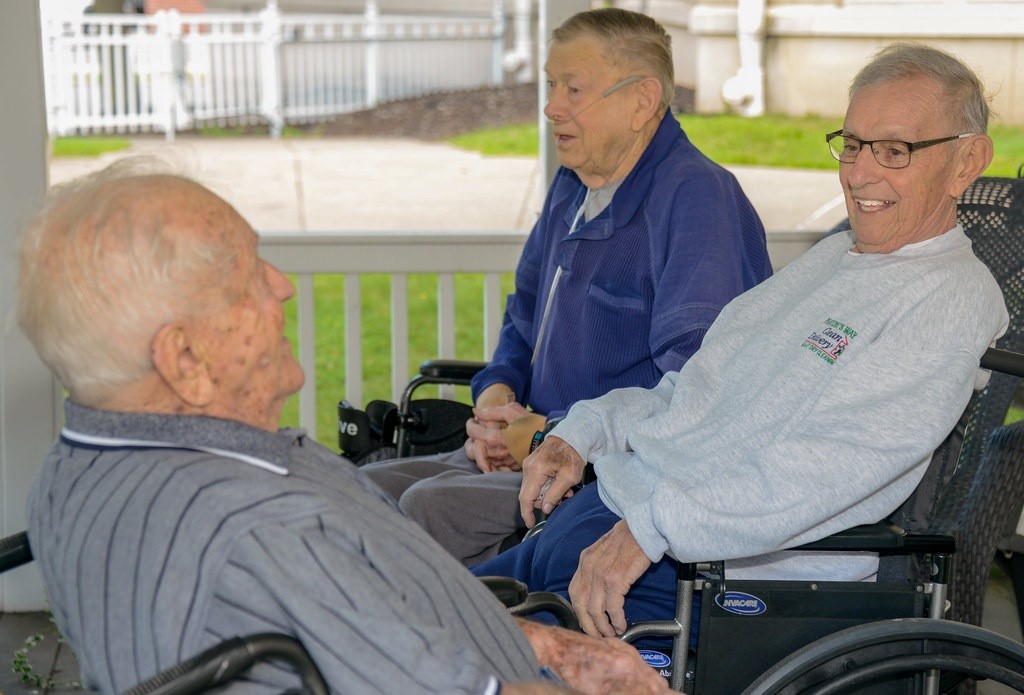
[349,7,785,582]
[439,34,1007,684]
[3,151,686,695]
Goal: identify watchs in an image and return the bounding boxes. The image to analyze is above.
[514,431,552,465]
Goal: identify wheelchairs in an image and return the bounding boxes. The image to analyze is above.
[527,178,1023,695]
[339,356,494,472]
[0,525,582,693]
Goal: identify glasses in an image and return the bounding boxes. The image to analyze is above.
[824,129,975,169]
